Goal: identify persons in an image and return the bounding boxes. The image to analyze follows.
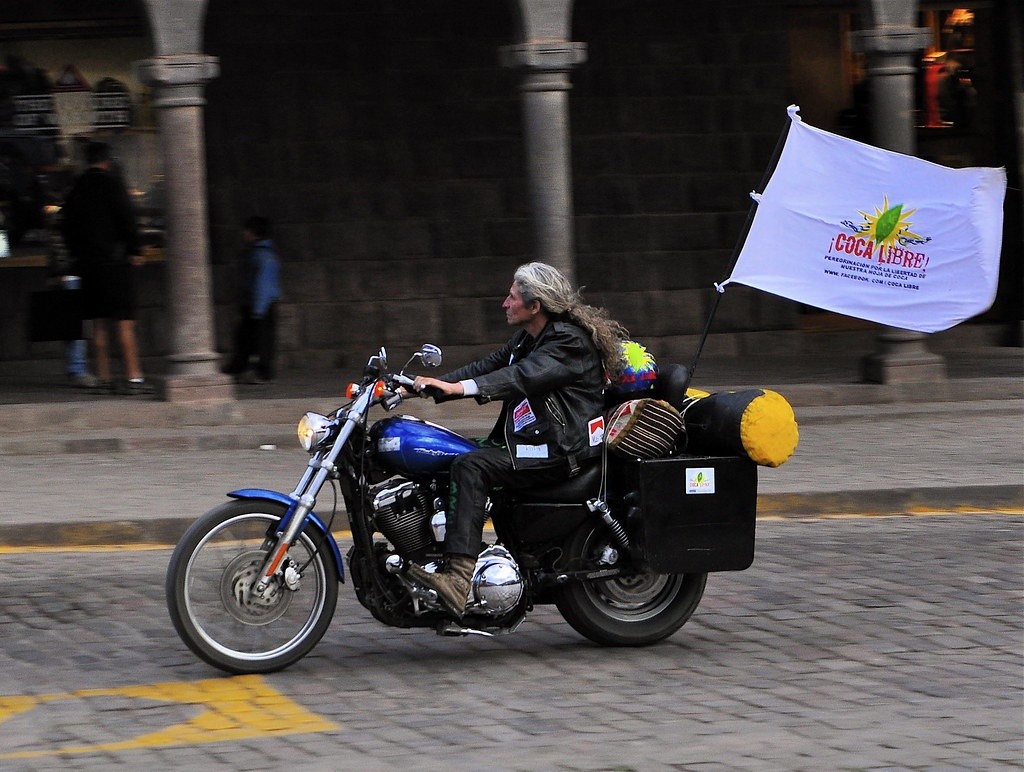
[392,262,630,624]
[222,217,284,385]
[16,157,101,393]
[59,140,157,394]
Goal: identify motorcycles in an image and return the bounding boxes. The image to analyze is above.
[159,341,759,676]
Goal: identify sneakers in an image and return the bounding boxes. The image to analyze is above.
[97,378,129,395]
[125,374,157,398]
[63,370,102,389]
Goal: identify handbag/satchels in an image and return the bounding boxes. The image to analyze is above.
[604,386,798,467]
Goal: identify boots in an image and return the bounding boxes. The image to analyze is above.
[409,555,475,619]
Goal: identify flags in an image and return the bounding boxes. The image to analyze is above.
[715,105,1008,336]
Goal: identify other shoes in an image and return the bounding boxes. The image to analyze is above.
[222,367,253,385]
[250,372,276,384]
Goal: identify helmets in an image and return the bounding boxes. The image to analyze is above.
[615,339,657,392]
